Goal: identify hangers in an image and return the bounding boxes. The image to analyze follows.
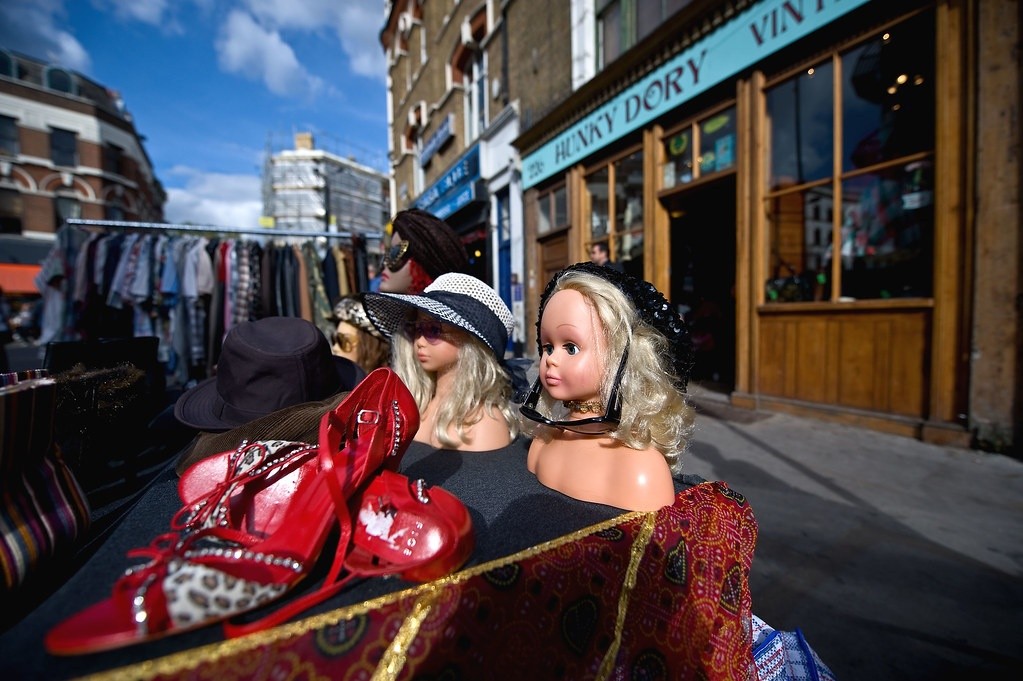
[69,217,386,253]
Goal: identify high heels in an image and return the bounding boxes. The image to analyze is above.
[43,370,476,655]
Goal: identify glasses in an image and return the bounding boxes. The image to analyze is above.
[520,327,634,435]
[331,332,365,353]
[379,240,418,272]
[402,319,450,343]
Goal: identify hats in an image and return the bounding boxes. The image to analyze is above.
[175,317,366,429]
[393,208,470,281]
[334,295,391,346]
[361,273,534,402]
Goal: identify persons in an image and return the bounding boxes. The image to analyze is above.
[526,260,695,513]
[378,208,470,294]
[591,242,621,272]
[359,274,521,452]
[332,293,392,375]
